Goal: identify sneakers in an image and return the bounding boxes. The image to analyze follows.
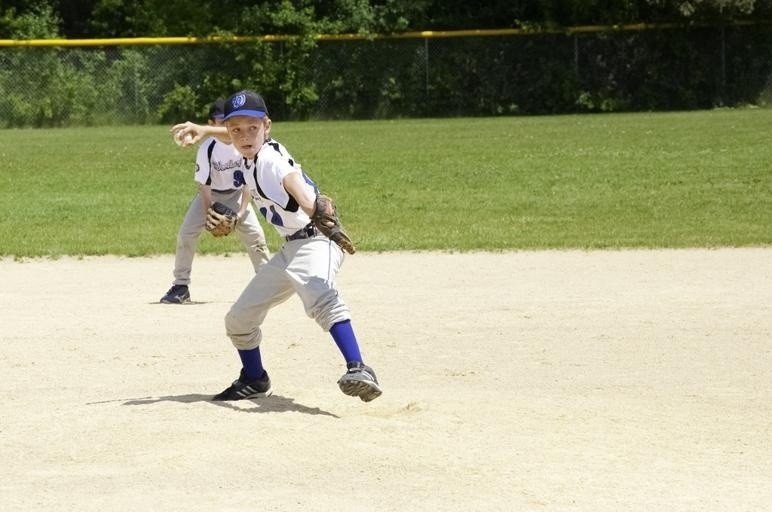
[160,286,190,304]
[339,360,383,402]
[211,367,273,400]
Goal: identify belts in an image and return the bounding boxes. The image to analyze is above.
[285,222,313,242]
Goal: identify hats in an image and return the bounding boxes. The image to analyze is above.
[210,90,270,122]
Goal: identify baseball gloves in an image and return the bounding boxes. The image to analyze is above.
[308,195,355,254]
[205,203,237,237]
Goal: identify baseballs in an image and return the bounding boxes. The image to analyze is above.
[173,130,192,147]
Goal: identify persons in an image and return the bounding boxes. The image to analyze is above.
[168,89,382,404]
[154,98,276,306]
